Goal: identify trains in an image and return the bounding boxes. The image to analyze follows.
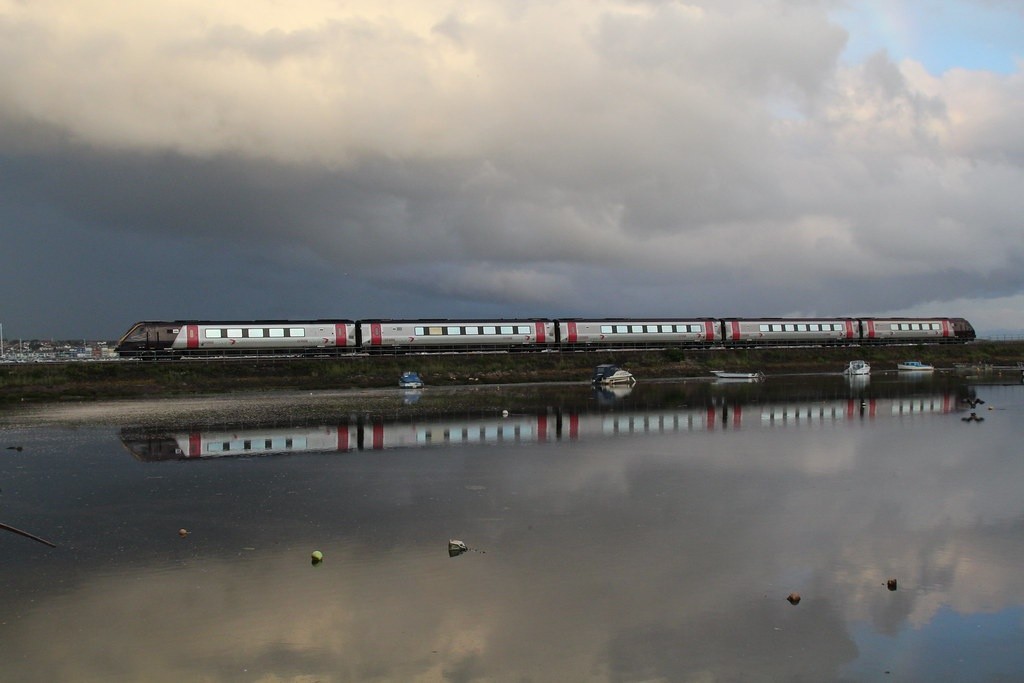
[113,316,979,357]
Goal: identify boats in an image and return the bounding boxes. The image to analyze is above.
[708,369,766,378]
[398,370,426,388]
[896,361,936,369]
[844,360,872,377]
[593,366,637,384]
